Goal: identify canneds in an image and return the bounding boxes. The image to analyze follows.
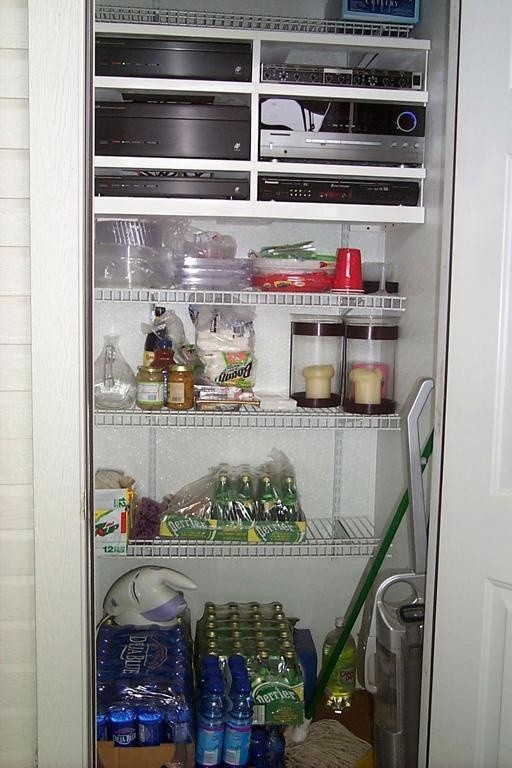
[136,364,166,409]
[167,364,195,410]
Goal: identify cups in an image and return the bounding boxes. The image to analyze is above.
[330,248,367,293]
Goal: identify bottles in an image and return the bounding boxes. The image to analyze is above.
[98,601,305,767]
[142,307,174,367]
[321,617,357,715]
[94,335,138,410]
[212,463,301,522]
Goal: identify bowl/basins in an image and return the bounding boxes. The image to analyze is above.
[254,257,334,292]
